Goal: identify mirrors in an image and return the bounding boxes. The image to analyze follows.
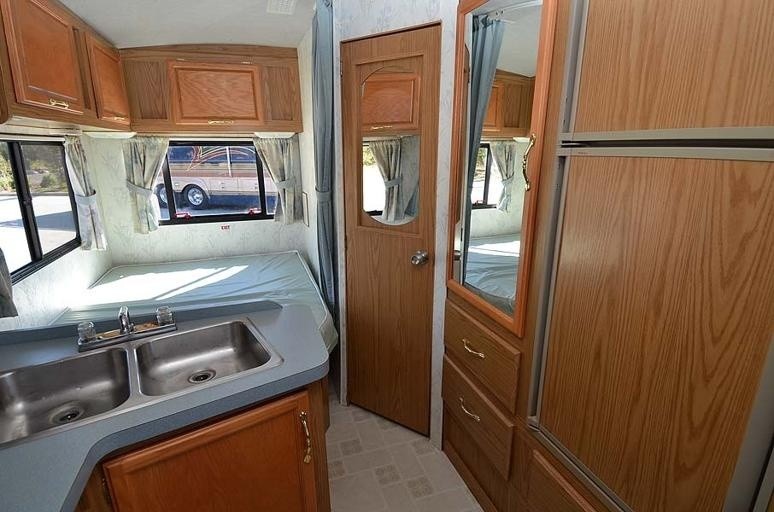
[354,65,421,225]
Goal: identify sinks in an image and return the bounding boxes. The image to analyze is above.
[0,347,131,451]
[134,316,284,400]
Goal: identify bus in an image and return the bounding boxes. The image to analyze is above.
[148,144,280,210]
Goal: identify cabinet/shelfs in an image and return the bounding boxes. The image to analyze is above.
[66,375,331,511]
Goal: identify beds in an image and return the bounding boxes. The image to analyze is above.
[49,250,338,434]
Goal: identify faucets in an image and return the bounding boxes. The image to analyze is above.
[118,306,134,336]
